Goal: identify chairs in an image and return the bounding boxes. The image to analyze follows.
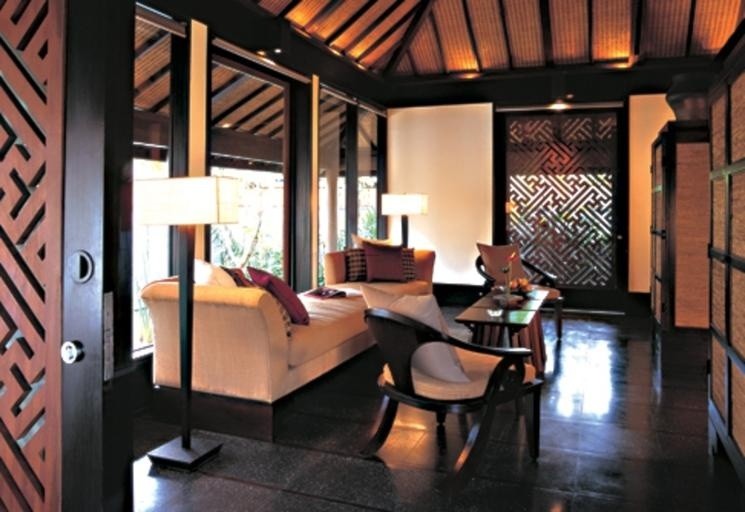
[346,309,545,498]
[476,253,564,338]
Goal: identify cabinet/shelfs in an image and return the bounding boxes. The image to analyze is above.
[707,18,745,489]
[650,121,710,342]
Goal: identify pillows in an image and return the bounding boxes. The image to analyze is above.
[193,260,310,325]
[344,233,415,283]
[360,284,473,383]
[476,240,527,285]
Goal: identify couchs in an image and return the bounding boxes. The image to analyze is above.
[140,245,435,442]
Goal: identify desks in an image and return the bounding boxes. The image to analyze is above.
[454,290,550,378]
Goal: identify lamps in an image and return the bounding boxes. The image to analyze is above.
[381,194,428,248]
[133,176,240,470]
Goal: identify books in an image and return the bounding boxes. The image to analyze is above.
[305,287,364,300]
